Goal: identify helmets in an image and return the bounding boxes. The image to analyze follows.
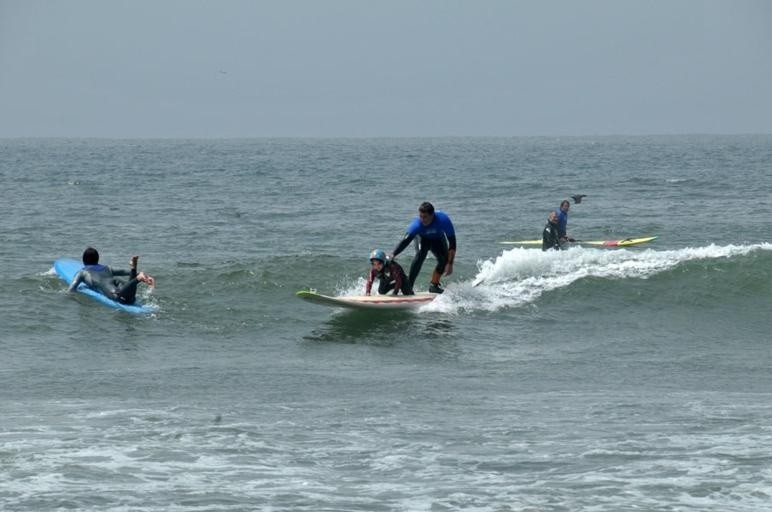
[369,249,387,265]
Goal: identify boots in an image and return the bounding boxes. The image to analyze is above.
[429,282,444,293]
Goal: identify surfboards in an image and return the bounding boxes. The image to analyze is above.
[295,290,441,311]
[54,256,160,314]
[497,236,658,247]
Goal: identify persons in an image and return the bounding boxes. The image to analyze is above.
[365,247,416,297]
[65,246,155,305]
[390,200,458,294]
[555,200,576,244]
[542,210,563,252]
